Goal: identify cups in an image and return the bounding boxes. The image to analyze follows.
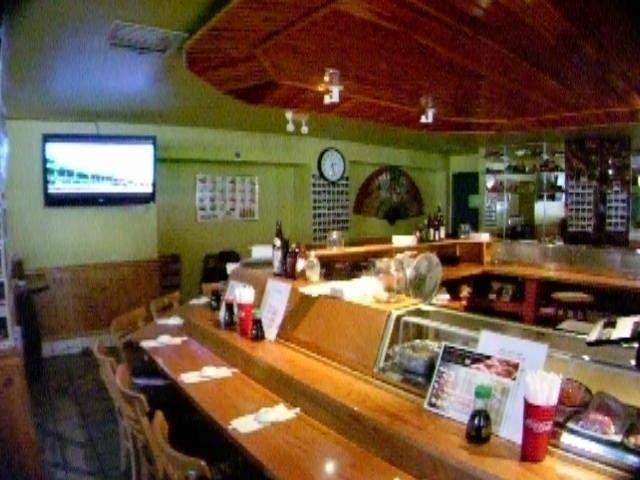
[520,397,558,463]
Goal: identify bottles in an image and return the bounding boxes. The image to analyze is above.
[466,384,493,444]
[416,206,446,242]
[326,230,344,251]
[272,221,320,282]
[210,287,265,341]
[458,224,471,239]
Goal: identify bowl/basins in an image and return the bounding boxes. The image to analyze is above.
[392,234,417,245]
[469,232,490,241]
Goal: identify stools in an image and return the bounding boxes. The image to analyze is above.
[550,289,594,327]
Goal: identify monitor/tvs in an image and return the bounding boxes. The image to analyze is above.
[41,132,157,207]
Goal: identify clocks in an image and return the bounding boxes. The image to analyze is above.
[318,147,345,183]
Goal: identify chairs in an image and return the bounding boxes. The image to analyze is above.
[92,290,216,480]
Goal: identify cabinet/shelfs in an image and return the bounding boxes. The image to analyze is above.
[484,139,567,240]
